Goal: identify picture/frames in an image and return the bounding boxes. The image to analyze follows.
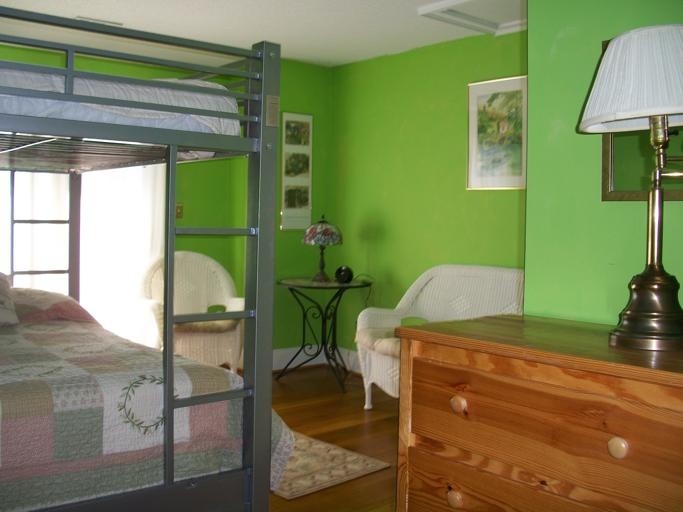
[466,73,527,192]
[600,39,683,203]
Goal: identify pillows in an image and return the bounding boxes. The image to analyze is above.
[0,272,99,325]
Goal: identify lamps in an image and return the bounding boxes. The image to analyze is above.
[304,213,343,282]
[578,23,682,352]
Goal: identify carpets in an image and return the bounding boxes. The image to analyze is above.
[272,430,393,502]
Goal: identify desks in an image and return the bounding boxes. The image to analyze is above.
[274,277,373,394]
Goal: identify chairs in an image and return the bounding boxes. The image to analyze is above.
[144,250,246,375]
[355,264,525,412]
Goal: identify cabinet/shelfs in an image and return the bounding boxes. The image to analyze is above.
[394,315,683,512]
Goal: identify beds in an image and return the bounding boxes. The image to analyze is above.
[0,6,283,512]
